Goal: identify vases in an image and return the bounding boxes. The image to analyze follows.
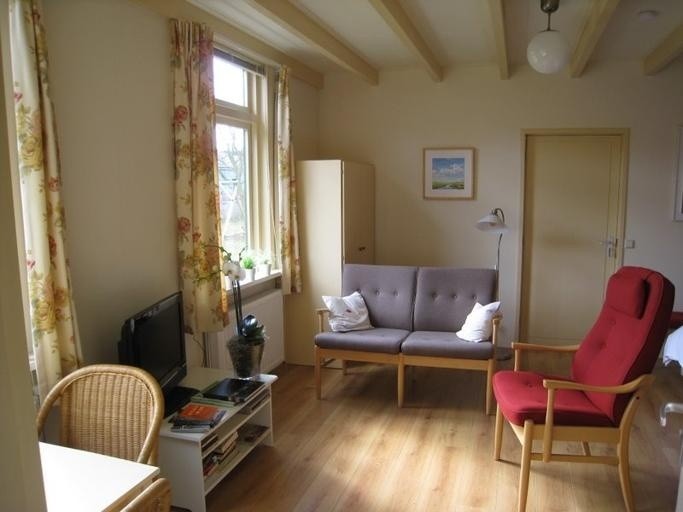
[225,334,266,378]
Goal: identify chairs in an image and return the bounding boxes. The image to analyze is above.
[311,265,502,417]
[488,265,675,512]
[121,477,171,511]
[37,360,167,474]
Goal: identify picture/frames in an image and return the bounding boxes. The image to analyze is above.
[423,145,478,202]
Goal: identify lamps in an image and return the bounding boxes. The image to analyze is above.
[472,205,516,364]
[524,1,575,76]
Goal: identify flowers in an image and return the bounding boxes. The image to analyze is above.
[188,240,250,343]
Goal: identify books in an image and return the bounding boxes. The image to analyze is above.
[167,376,271,482]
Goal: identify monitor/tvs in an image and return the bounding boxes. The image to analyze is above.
[118,289,199,419]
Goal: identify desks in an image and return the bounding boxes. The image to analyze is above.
[40,437,161,512]
[159,365,280,512]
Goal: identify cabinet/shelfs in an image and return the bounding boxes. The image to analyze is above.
[283,158,376,370]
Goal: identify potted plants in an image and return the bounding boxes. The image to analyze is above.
[261,260,272,276]
[243,256,256,282]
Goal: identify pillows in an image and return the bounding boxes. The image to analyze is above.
[320,290,374,333]
[455,301,502,343]
